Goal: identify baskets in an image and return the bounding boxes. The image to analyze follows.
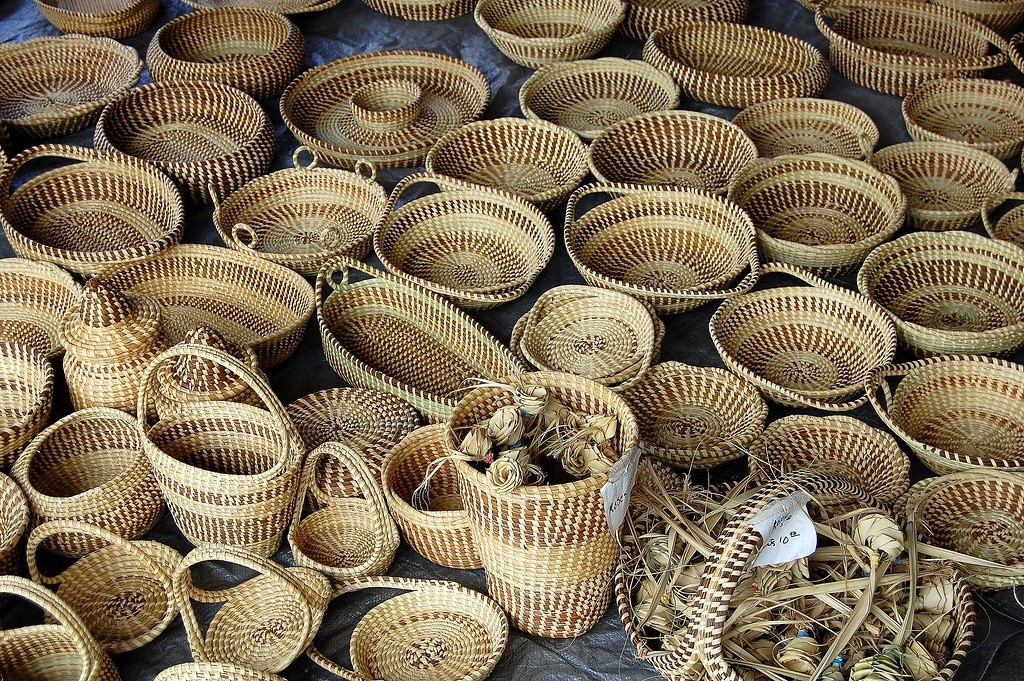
[0,0,1024,681]
